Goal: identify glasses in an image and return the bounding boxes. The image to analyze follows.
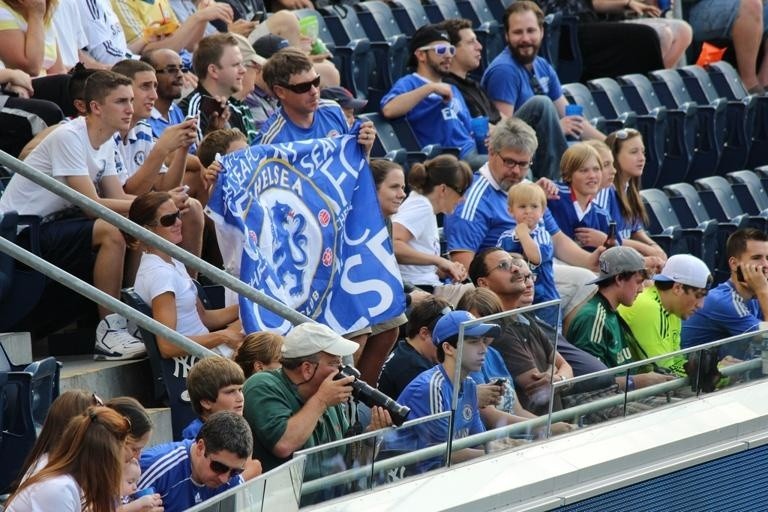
[521,272,539,283]
[147,209,181,227]
[495,152,533,168]
[414,44,456,57]
[611,128,639,144]
[201,433,246,477]
[445,182,468,195]
[155,68,189,74]
[427,304,454,329]
[278,73,321,94]
[484,259,522,280]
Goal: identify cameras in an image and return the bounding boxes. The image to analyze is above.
[330,361,411,429]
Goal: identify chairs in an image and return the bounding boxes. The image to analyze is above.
[0,355,63,502]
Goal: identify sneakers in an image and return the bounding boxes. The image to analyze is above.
[93,313,146,361]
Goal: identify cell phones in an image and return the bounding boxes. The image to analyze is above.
[734,265,766,282]
[180,113,197,124]
[197,95,222,115]
[491,376,508,388]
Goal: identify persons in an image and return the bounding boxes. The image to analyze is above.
[0,2,767,512]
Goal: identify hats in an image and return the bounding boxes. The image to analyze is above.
[320,86,369,109]
[279,321,360,359]
[583,246,646,286]
[411,23,452,53]
[650,254,713,290]
[431,310,502,348]
[251,33,289,59]
